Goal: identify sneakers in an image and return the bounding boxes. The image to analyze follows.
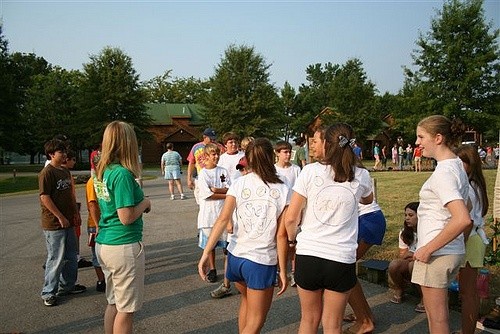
[210,283,232,298]
[59,284,86,293]
[44,295,58,306]
[206,269,217,283]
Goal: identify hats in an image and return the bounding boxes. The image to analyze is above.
[204,128,215,136]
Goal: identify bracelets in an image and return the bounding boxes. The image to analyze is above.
[288,240,297,245]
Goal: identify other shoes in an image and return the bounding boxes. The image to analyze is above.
[275,271,280,287]
[290,271,296,286]
[78,258,93,269]
[96,280,106,291]
[180,196,184,199]
[170,195,175,200]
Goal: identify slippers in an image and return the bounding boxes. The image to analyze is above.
[343,313,375,324]
[415,304,426,312]
[390,294,403,303]
[341,327,372,334]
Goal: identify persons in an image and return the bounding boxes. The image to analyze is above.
[61,149,86,267]
[186,127,309,299]
[411,115,473,334]
[39,138,87,306]
[372,136,500,173]
[453,145,488,334]
[310,128,386,334]
[84,140,143,292]
[42,135,67,269]
[95,121,151,334]
[388,202,427,312]
[285,122,373,334]
[198,139,288,334]
[161,143,187,201]
[194,143,232,283]
